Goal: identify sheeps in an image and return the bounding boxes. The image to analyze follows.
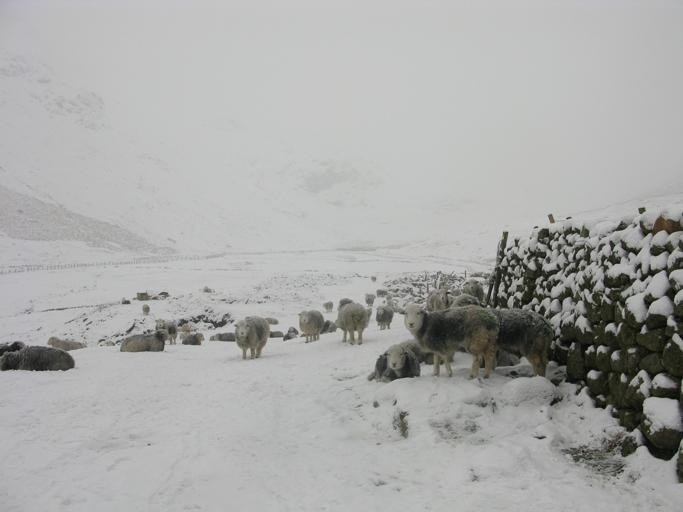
[324,302,333,313]
[0,337,87,371]
[366,276,554,382]
[234,316,269,359]
[120,319,204,352]
[265,309,337,343]
[338,298,369,345]
